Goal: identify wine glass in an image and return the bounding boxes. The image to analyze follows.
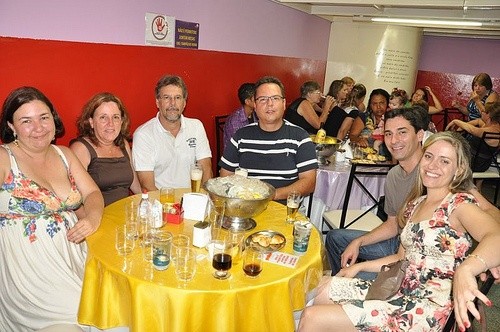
[320,94,337,104]
[212,242,234,281]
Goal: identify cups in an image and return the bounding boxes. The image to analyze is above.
[169,236,190,262]
[125,200,139,222]
[227,227,246,265]
[175,247,197,282]
[284,190,301,224]
[115,225,136,255]
[208,239,225,261]
[292,216,312,257]
[190,164,203,193]
[209,199,226,240]
[354,146,363,159]
[234,168,249,178]
[242,247,264,279]
[159,187,175,205]
[142,229,173,271]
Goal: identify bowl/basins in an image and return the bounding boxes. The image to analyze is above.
[362,147,378,155]
[308,134,343,166]
[204,177,276,232]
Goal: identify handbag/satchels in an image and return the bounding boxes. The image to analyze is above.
[364,259,406,302]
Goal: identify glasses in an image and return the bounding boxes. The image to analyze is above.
[315,90,323,95]
[481,111,487,115]
[255,94,285,104]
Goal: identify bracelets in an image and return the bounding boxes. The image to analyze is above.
[468,253,488,272]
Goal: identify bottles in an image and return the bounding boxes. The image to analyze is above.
[315,121,327,144]
[136,193,151,246]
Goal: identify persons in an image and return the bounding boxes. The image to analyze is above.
[223,83,257,154]
[218,76,320,206]
[285,80,335,134]
[68,92,143,222]
[445,101,500,172]
[452,72,500,127]
[132,75,212,191]
[326,106,500,281]
[0,86,129,332]
[313,77,444,161]
[297,130,500,332]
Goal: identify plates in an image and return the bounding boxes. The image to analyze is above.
[349,156,386,166]
[205,243,223,253]
[244,229,287,254]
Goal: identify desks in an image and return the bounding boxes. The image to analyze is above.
[311,147,387,247]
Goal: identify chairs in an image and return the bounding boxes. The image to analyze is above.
[443,107,466,132]
[471,130,500,177]
[299,195,328,233]
[320,160,399,238]
[444,269,497,332]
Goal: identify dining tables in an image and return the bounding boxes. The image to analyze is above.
[75,186,326,332]
